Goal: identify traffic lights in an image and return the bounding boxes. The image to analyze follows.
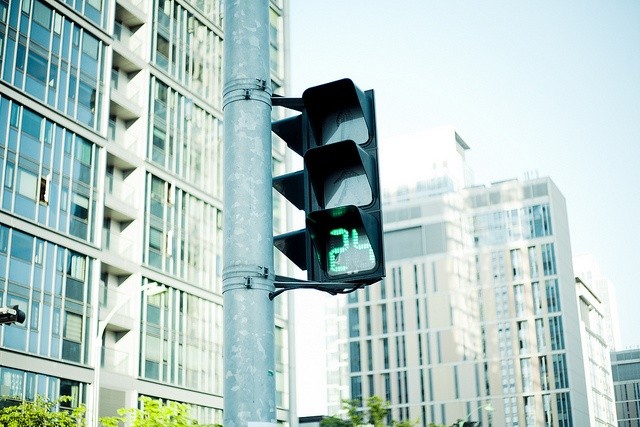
[301,76,385,282]
[271,112,308,281]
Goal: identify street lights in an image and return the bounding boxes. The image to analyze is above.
[92,282,167,427]
[459,403,496,427]
